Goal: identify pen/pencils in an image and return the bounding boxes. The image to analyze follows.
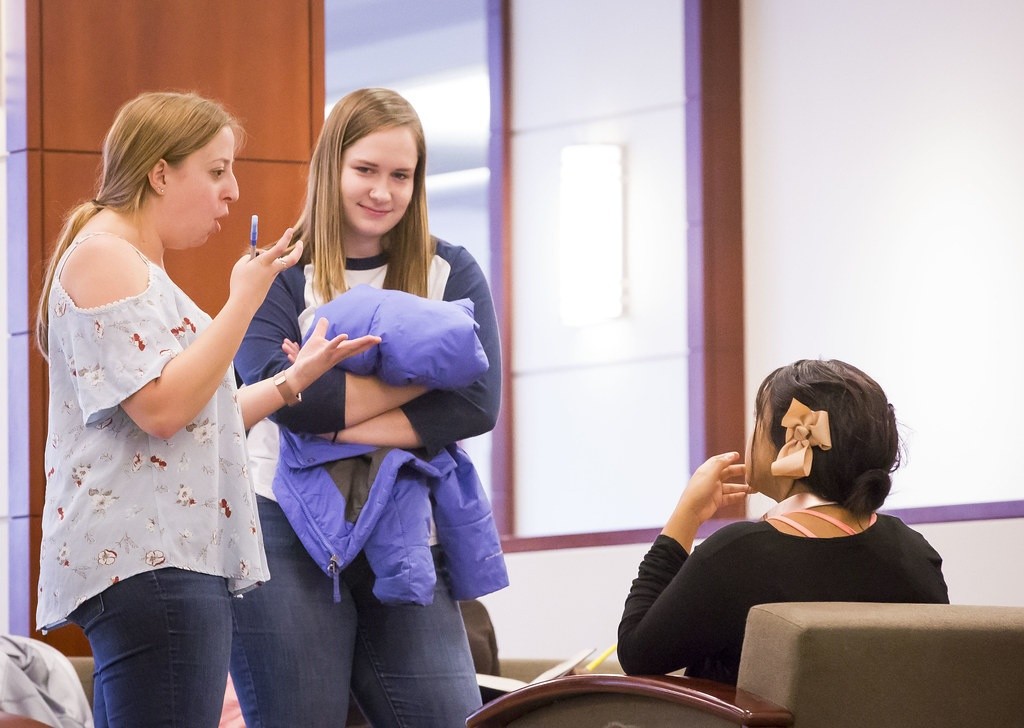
[250,214,259,260]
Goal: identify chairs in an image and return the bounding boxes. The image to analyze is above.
[464,599,1023,728]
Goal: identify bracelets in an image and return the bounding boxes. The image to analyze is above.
[274,370,302,408]
[332,430,338,444]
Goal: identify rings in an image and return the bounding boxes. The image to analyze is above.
[281,258,287,271]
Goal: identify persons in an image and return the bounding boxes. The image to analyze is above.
[230,87,504,728]
[33,91,382,727]
[617,358,950,691]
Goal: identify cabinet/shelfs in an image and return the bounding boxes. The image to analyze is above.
[27,0,328,659]
